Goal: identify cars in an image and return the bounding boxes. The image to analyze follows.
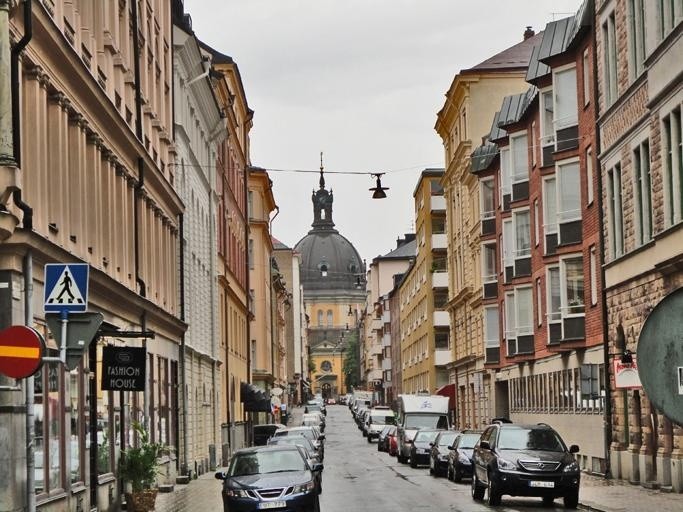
[19,423,168,499]
[338,388,484,484]
[327,399,337,405]
[213,391,327,511]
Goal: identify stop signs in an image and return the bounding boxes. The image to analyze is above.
[0,324,40,379]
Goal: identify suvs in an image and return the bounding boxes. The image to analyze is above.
[470,417,580,510]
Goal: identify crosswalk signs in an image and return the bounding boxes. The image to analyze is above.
[42,262,88,313]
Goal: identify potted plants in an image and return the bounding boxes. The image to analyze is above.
[116,420,177,512]
